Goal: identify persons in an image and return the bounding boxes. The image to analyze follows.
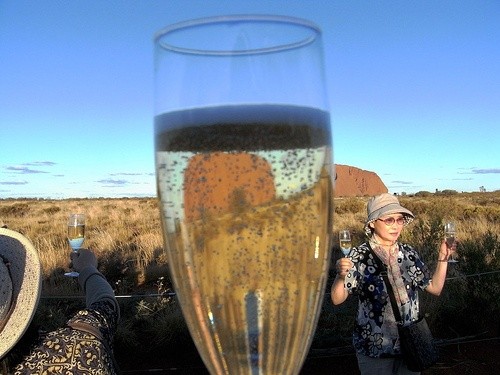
[0,213,121,375]
[331,195,458,375]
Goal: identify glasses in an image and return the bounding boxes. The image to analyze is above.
[374,217,404,226]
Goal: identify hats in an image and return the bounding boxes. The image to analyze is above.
[0,228,41,361]
[363,193,415,237]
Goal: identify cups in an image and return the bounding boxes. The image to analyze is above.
[153,14,334,375]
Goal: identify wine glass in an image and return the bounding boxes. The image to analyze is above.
[64,214,86,277]
[339,230,354,272]
[445,221,458,263]
